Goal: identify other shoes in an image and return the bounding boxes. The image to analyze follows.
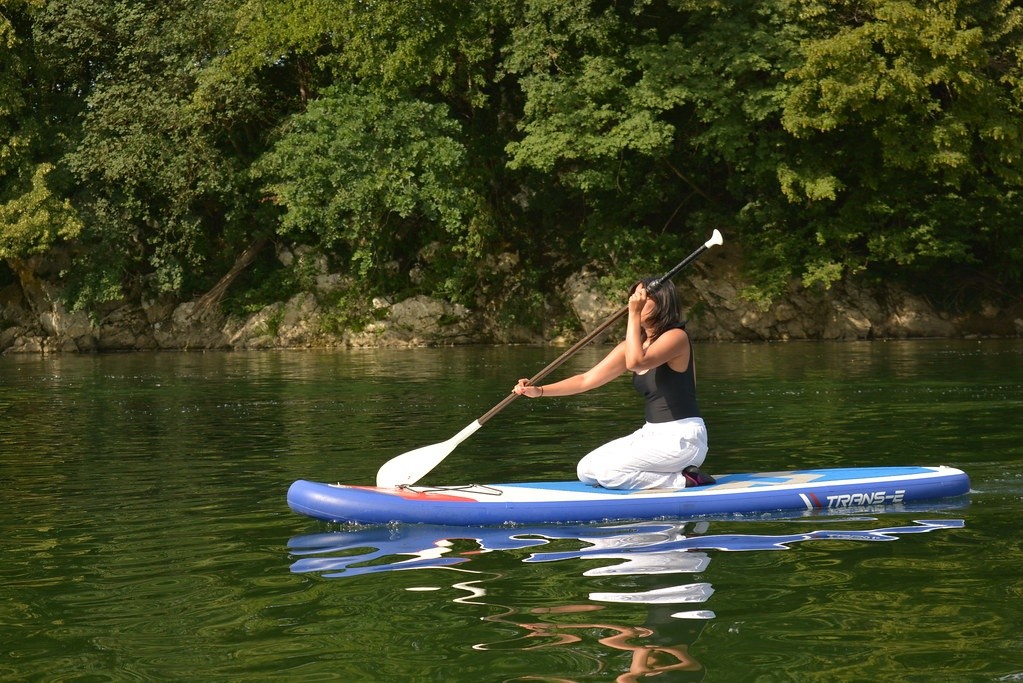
[682,465,716,486]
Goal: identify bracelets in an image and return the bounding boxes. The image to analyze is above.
[539,386,543,398]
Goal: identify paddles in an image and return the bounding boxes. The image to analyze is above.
[376,228,725,487]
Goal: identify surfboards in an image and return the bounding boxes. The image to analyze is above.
[284,464,973,528]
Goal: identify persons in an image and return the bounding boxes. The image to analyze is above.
[511,276,716,489]
